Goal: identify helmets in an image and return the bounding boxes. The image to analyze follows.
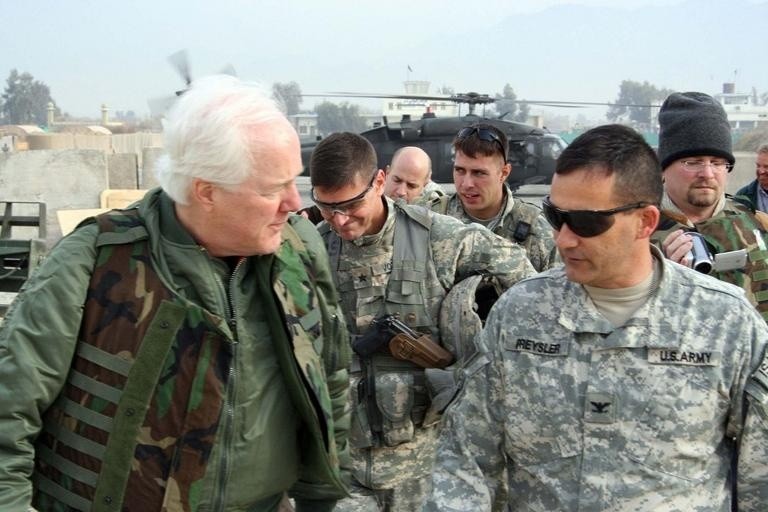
[440,274,503,362]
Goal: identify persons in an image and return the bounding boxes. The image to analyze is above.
[424,125,768,512]
[1,74,353,512]
[735,144,767,211]
[313,131,538,512]
[381,146,444,207]
[425,125,563,274]
[647,91,768,319]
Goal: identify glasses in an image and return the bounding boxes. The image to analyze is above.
[679,160,733,172]
[457,127,507,165]
[542,195,660,238]
[310,168,379,217]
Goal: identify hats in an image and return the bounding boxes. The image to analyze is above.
[659,92,735,172]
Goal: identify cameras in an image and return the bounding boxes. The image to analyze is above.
[681,230,749,276]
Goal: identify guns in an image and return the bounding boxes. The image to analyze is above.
[351,311,452,371]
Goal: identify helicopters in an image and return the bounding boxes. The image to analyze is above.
[166,50,662,186]
